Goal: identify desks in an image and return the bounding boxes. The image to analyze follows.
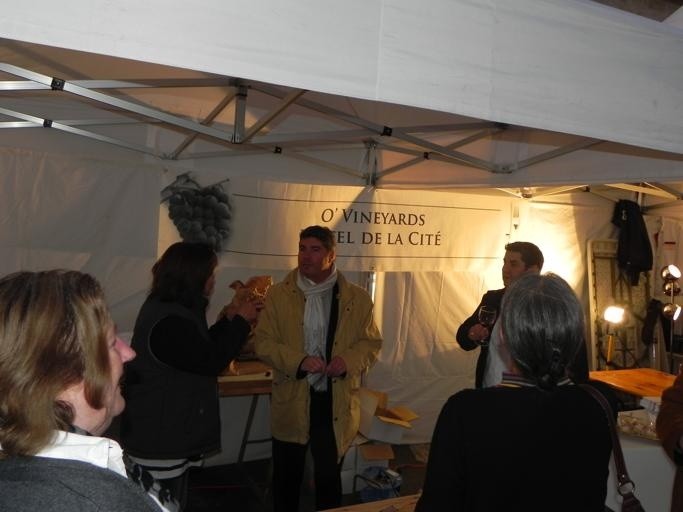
[217,381,274,473]
[319,492,422,512]
[587,367,678,512]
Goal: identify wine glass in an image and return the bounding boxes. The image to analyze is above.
[476,304,497,346]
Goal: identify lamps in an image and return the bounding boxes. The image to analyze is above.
[660,263,682,375]
[604,304,643,368]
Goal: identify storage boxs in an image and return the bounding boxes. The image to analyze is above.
[355,386,419,469]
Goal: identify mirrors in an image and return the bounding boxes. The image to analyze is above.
[586,238,657,409]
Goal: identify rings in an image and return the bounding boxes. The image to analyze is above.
[469,331,473,336]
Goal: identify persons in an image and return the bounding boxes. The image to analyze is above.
[252,223,385,511]
[121,241,265,511]
[656,368,683,511]
[412,271,619,511]
[453,242,542,390]
[0,270,182,512]
